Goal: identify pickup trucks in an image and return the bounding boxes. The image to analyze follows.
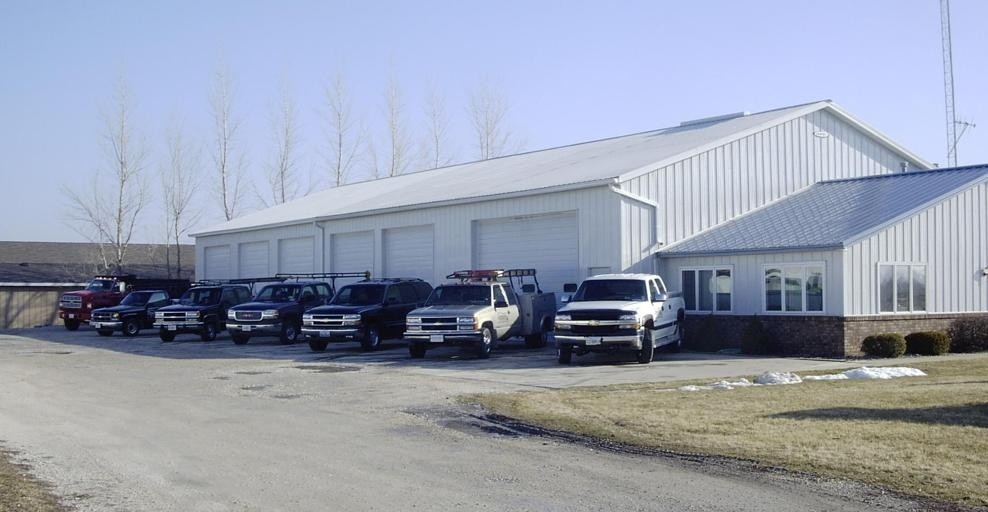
[399,263,554,358]
[549,271,691,362]
[89,287,180,336]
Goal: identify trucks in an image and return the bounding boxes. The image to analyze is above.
[56,271,191,332]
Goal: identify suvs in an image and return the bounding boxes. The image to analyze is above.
[151,276,254,342]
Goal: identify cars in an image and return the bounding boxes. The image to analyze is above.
[298,276,435,352]
[228,281,333,344]
